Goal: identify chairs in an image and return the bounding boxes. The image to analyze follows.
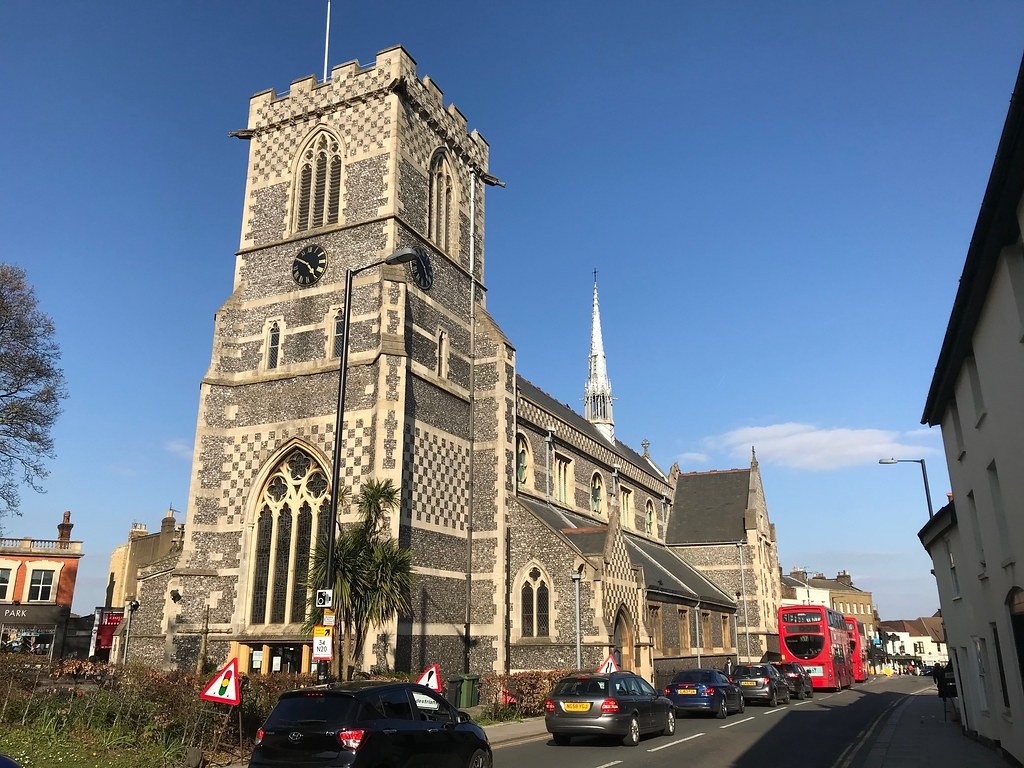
[587,682,601,693]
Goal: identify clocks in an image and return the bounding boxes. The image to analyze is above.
[409,247,434,290]
[291,244,329,287]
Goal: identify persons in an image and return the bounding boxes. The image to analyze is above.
[724,657,736,676]
[933,663,950,698]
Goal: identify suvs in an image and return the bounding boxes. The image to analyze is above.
[767,661,814,700]
[247,679,494,768]
[543,668,676,746]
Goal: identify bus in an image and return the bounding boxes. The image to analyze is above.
[777,604,855,692]
[843,617,871,683]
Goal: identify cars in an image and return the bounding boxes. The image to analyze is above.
[920,666,933,676]
[663,667,745,719]
[729,663,791,707]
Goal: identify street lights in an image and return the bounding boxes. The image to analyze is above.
[570,568,583,671]
[736,542,752,664]
[879,457,934,519]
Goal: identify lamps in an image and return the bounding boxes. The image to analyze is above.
[170,589,182,602]
